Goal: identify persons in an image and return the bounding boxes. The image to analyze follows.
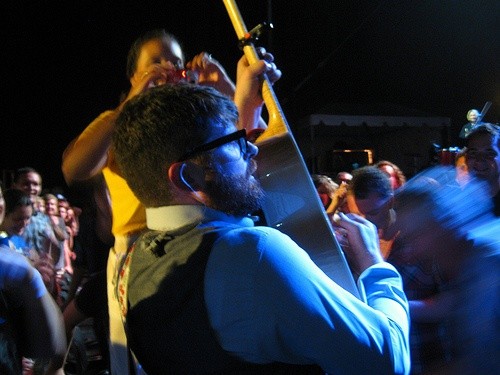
[0,168,115,375]
[62,28,268,375]
[110,47,409,375]
[311,109,500,375]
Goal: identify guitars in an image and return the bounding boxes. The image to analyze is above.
[222,0,363,303]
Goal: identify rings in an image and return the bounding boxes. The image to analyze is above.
[144,70,149,76]
[208,54,213,60]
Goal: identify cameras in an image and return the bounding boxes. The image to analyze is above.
[164,68,200,86]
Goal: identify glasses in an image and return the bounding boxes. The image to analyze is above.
[159,128,250,190]
